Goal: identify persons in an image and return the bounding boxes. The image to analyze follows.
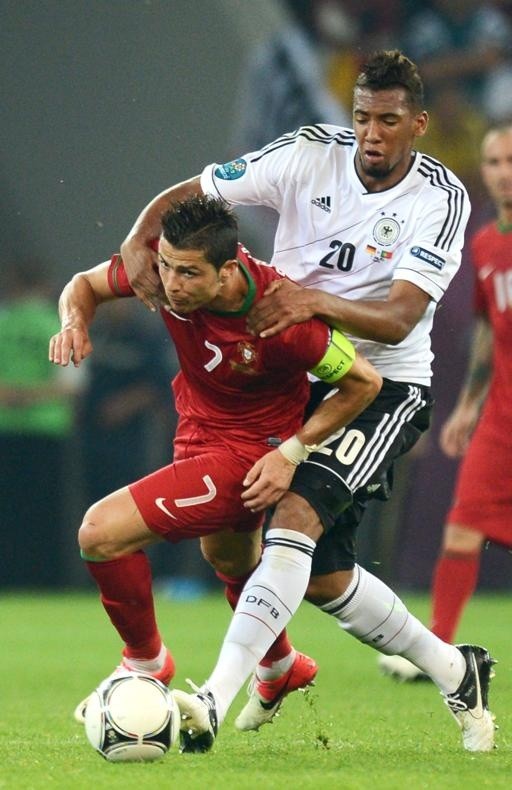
[0,259,221,596]
[47,195,385,731]
[375,124,512,686]
[118,46,502,756]
[291,0,512,598]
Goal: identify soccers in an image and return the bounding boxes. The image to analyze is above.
[85,672,180,761]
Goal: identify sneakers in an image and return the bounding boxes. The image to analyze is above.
[438,644,499,752]
[380,654,431,683]
[235,650,318,731]
[73,648,175,724]
[172,677,218,754]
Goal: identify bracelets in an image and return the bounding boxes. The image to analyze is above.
[277,435,309,466]
